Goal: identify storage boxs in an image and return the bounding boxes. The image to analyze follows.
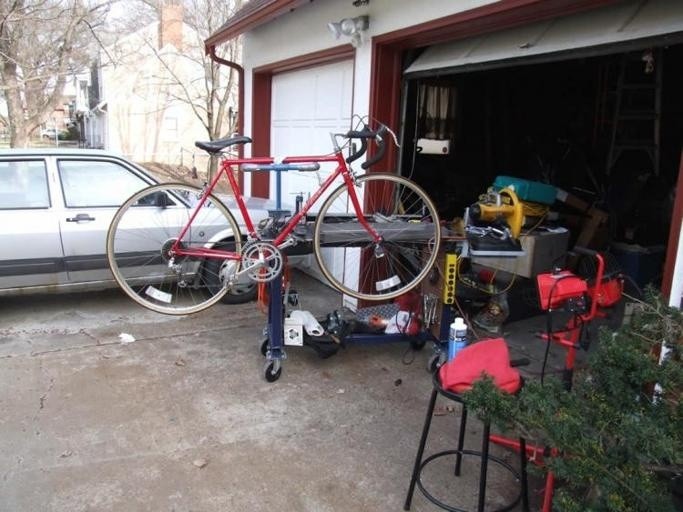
[468,227,569,279]
[612,241,665,298]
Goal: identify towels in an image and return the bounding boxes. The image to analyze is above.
[439,337,521,395]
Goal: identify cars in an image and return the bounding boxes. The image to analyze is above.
[2,151,274,303]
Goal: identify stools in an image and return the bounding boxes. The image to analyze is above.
[404,359,530,512]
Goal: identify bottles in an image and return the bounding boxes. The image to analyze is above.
[448,316,468,363]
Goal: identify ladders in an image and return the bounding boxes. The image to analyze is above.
[607,50,661,208]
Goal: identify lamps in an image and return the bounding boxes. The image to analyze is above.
[327,15,369,49]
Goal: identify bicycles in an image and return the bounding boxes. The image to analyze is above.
[105,116,441,315]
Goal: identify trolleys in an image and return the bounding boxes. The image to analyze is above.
[261,210,459,382]
